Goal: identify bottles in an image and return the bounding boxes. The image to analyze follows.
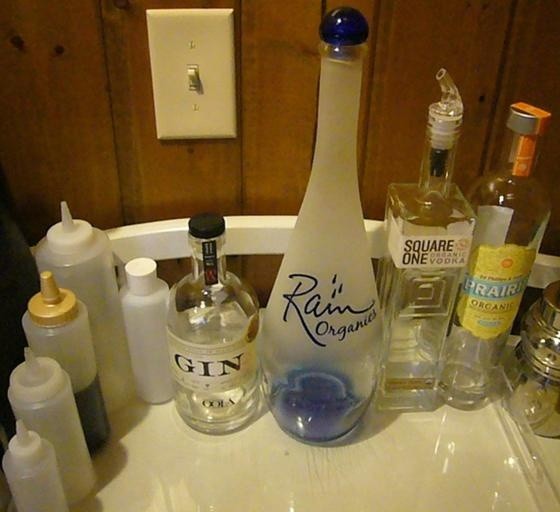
[374,69,481,414]
[17,271,115,457]
[167,209,261,436]
[119,256,176,406]
[502,277,560,441]
[438,94,560,414]
[7,346,100,504]
[262,5,382,446]
[2,419,70,512]
[33,194,135,419]
